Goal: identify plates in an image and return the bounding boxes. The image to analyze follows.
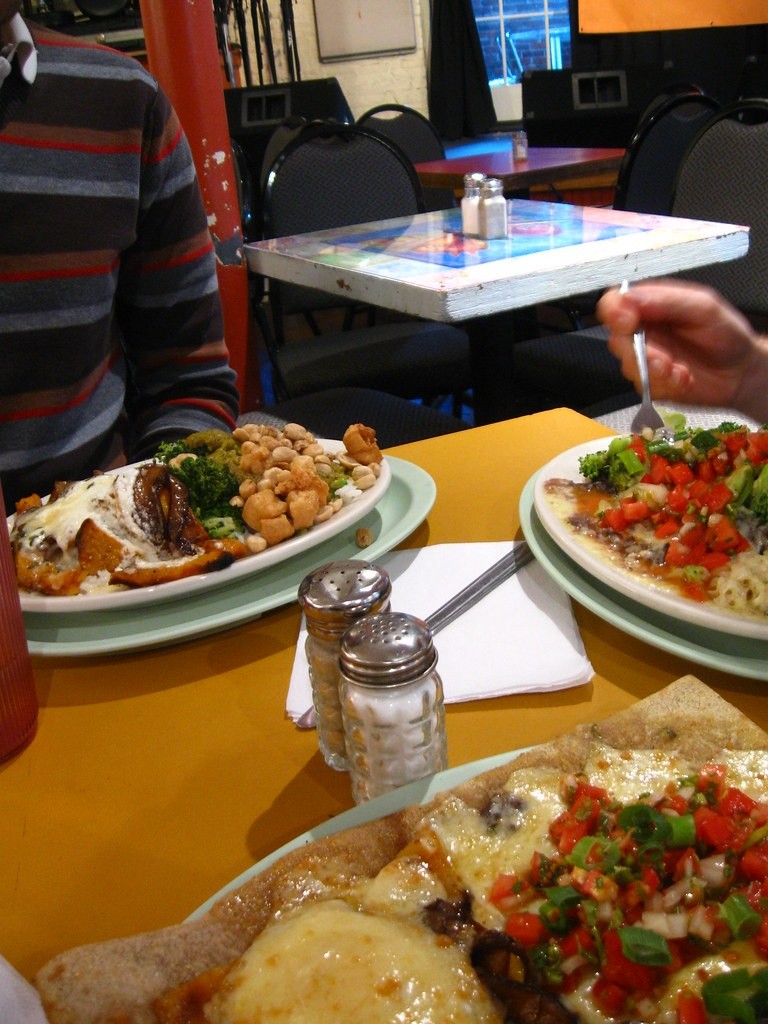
[177,749,533,923]
[531,432,768,639]
[518,468,767,680]
[3,436,391,612]
[28,455,437,658]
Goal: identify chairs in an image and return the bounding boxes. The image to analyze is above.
[616,92,727,215]
[235,386,472,451]
[260,119,469,390]
[358,104,445,171]
[514,98,768,423]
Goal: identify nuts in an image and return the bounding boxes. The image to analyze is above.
[230,423,382,546]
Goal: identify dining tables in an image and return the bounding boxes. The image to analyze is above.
[3,408,768,1024]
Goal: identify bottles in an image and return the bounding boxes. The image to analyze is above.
[1,501,39,764]
[461,171,487,236]
[477,179,507,241]
[337,611,448,801]
[512,131,528,161]
[297,558,394,771]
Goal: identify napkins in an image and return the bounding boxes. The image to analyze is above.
[285,542,596,723]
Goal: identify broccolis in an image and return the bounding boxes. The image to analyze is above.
[154,437,251,539]
[579,411,768,537]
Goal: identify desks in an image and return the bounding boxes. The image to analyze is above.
[243,198,752,406]
[409,148,623,205]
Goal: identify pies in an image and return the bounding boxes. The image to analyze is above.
[35,673,768,1024]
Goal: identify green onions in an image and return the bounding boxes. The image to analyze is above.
[506,768,768,1024]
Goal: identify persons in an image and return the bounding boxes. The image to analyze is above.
[597,282,768,426]
[0,0,240,518]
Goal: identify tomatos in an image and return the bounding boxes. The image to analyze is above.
[600,425,768,603]
